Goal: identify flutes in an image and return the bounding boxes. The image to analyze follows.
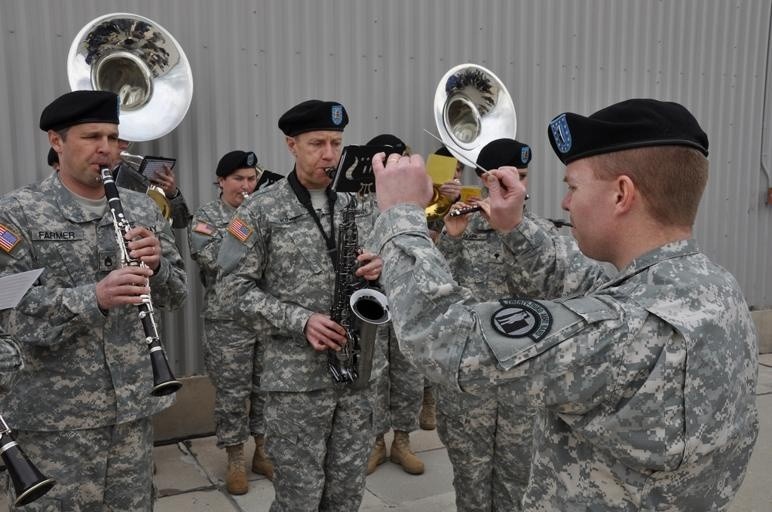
[450,195,531,219]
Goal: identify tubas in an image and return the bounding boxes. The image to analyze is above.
[66,12,192,224]
[430,62,518,171]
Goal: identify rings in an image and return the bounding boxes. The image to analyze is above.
[374,269,378,273]
[386,158,398,163]
[152,246,156,255]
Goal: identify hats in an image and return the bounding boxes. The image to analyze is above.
[216,150,258,177]
[546,98,710,166]
[475,137,533,178]
[365,134,464,170]
[47,146,59,166]
[38,90,120,132]
[276,98,350,137]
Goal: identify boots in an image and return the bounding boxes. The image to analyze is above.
[389,430,425,475]
[366,433,387,475]
[419,388,437,430]
[251,430,278,483]
[225,443,249,496]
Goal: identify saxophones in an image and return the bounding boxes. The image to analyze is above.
[322,165,391,384]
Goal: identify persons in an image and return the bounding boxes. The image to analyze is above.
[418,146,483,433]
[215,99,382,511]
[47,146,61,173]
[0,90,188,511]
[359,134,464,475]
[112,139,189,231]
[427,139,564,512]
[370,97,759,511]
[187,149,276,496]
